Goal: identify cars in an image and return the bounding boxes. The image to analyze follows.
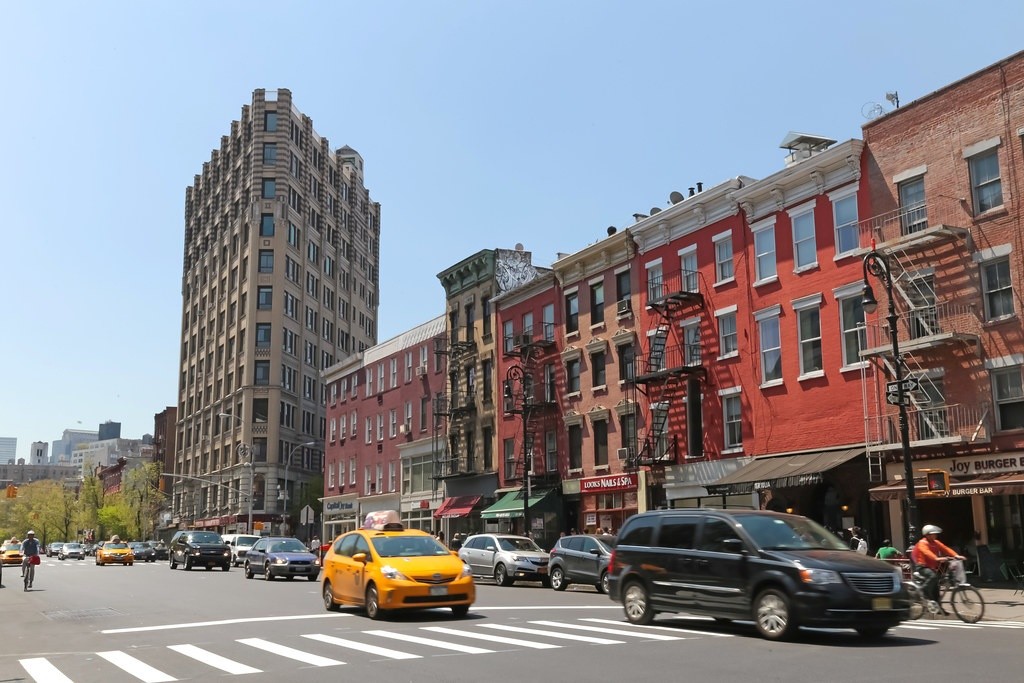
[0,530,266,582]
[242,537,321,582]
[318,510,474,617]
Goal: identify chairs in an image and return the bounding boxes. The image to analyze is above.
[1005,559,1024,596]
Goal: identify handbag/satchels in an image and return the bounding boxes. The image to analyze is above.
[28,555,40,565]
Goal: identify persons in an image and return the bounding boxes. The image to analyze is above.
[910,525,967,615]
[792,523,868,556]
[311,536,321,556]
[874,539,903,564]
[429,526,618,551]
[20,530,39,588]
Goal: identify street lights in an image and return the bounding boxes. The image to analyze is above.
[217,412,258,533]
[282,440,316,536]
[8,485,19,498]
[860,248,920,553]
[503,363,535,539]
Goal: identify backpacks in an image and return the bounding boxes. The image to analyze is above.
[852,536,867,556]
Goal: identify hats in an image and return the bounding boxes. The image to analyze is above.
[27,530,34,535]
[921,524,943,536]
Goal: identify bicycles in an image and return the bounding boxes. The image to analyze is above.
[895,556,986,623]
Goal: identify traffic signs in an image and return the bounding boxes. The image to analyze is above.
[886,379,918,392]
[886,393,910,407]
[926,474,946,491]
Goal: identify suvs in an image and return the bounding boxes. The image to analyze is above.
[454,529,554,587]
[546,534,620,593]
[606,507,914,637]
